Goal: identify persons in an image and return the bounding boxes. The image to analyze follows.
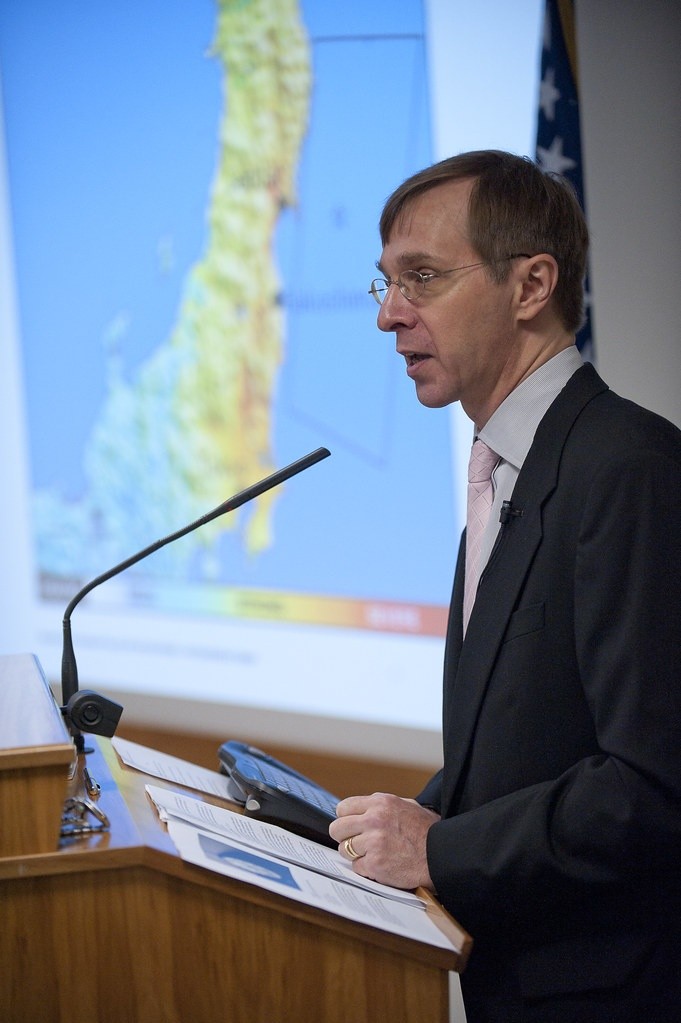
[328,150,681,1023]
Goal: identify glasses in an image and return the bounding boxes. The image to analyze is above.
[367,251,532,307]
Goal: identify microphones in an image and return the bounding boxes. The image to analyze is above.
[51,445,332,755]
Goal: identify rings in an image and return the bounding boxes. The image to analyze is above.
[344,837,362,858]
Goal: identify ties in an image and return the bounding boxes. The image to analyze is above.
[461,438,502,640]
